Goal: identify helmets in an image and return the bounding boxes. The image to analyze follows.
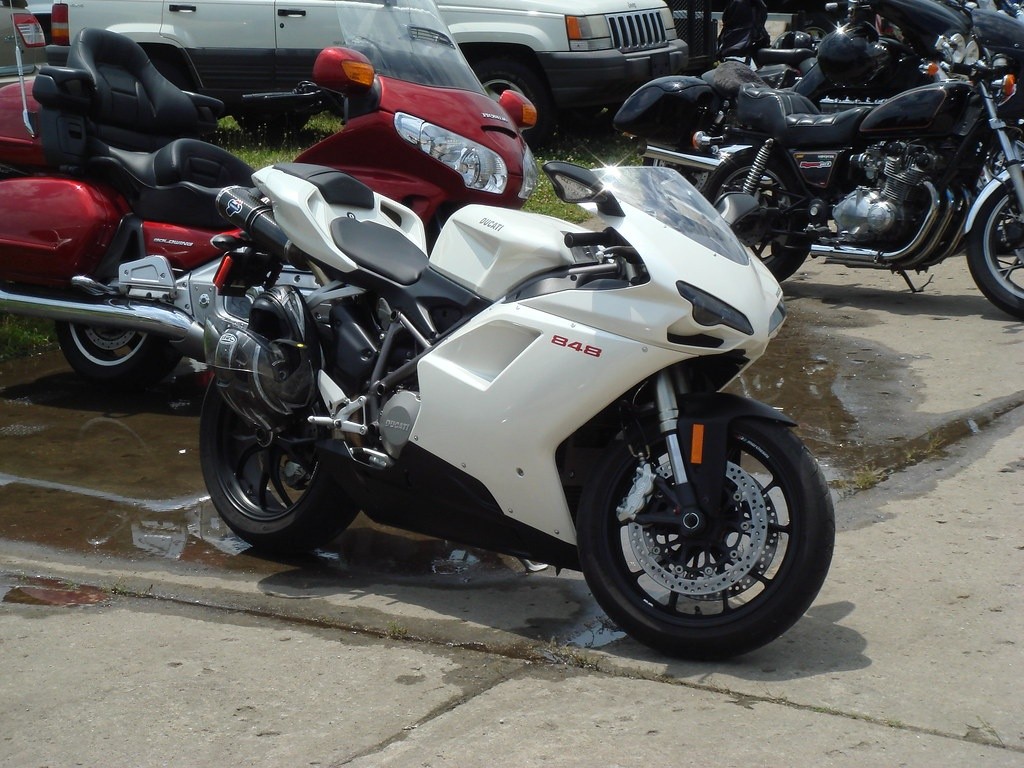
[817,28,890,89]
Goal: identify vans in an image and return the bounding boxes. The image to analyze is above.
[65,1,691,148]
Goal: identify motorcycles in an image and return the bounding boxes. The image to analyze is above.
[1,0,538,398]
[608,1,1024,322]
[195,164,836,663]
